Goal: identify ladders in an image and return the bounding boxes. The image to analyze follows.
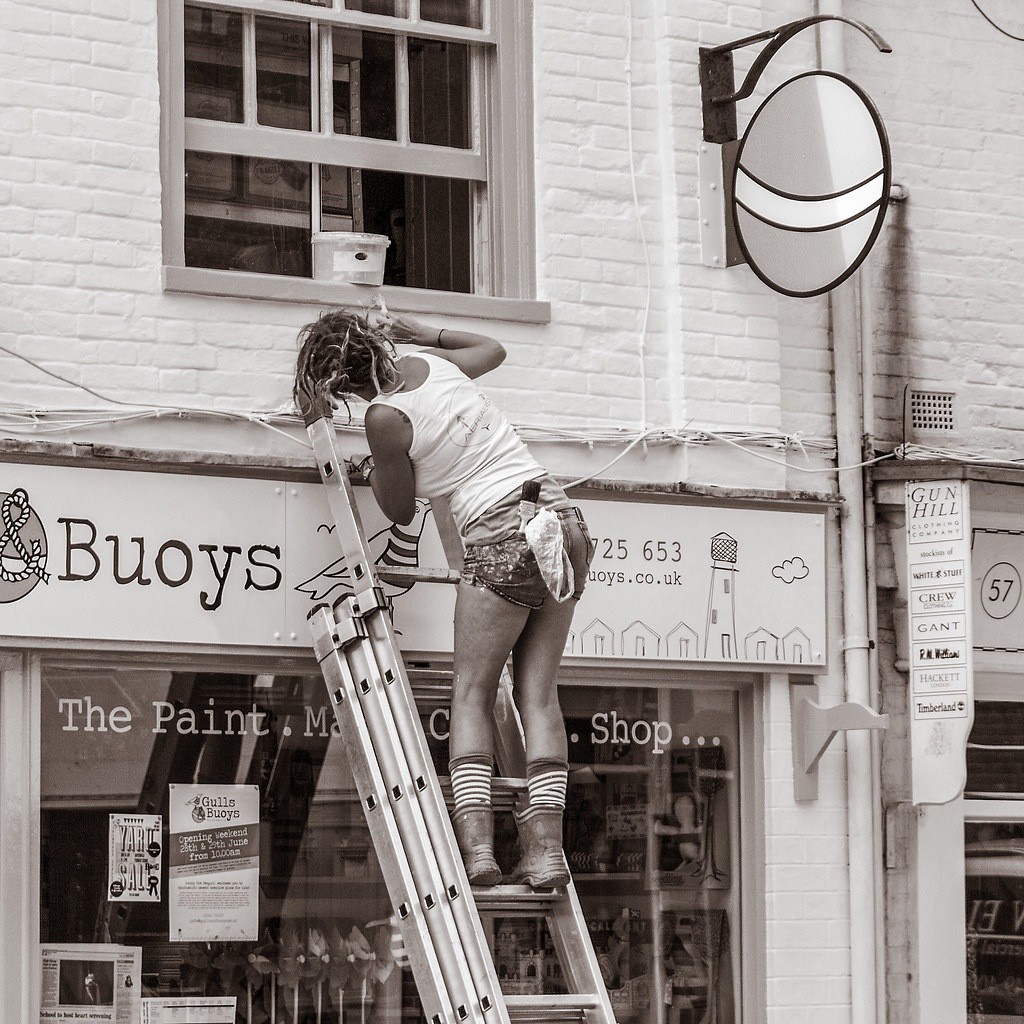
[286,416,627,1024]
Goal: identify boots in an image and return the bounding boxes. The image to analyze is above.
[452,803,504,886]
[508,807,571,889]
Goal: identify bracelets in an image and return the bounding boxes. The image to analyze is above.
[356,455,373,469]
[437,329,445,348]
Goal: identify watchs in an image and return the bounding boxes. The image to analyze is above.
[362,464,375,480]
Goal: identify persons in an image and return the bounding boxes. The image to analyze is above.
[294,306,595,889]
[86,968,95,980]
[125,975,133,987]
